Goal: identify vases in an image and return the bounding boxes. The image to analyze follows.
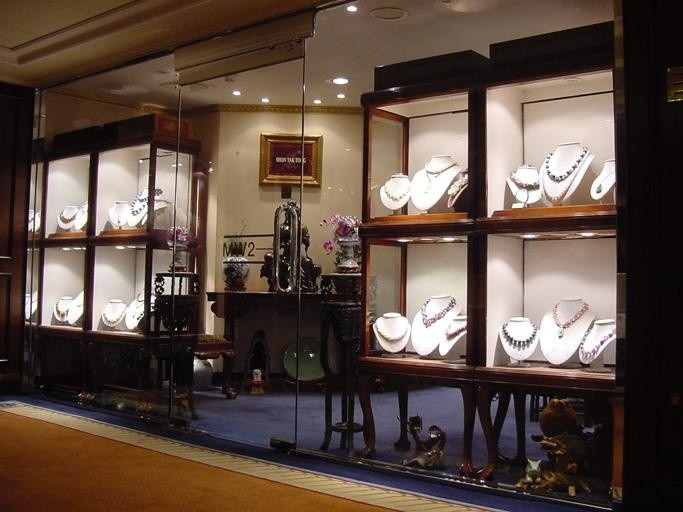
[336,240,359,272]
[169,244,188,269]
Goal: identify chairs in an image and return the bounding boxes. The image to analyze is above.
[153,270,238,419]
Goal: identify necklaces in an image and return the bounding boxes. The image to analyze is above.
[447,176,469,198]
[552,302,591,339]
[55,300,70,319]
[29,217,35,222]
[579,326,618,362]
[541,146,591,204]
[102,292,158,323]
[510,168,538,190]
[114,188,163,225]
[445,321,468,341]
[421,296,456,328]
[424,158,456,195]
[502,321,538,351]
[375,318,410,343]
[60,209,78,223]
[384,180,412,202]
[596,169,615,194]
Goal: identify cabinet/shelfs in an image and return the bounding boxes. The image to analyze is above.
[22,132,200,419]
[355,62,616,488]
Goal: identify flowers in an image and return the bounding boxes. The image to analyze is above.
[319,212,363,256]
[166,224,198,249]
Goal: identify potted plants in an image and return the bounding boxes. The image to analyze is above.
[220,220,251,291]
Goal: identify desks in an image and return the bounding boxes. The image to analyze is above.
[205,290,395,396]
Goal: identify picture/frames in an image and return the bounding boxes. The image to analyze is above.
[257,131,323,191]
